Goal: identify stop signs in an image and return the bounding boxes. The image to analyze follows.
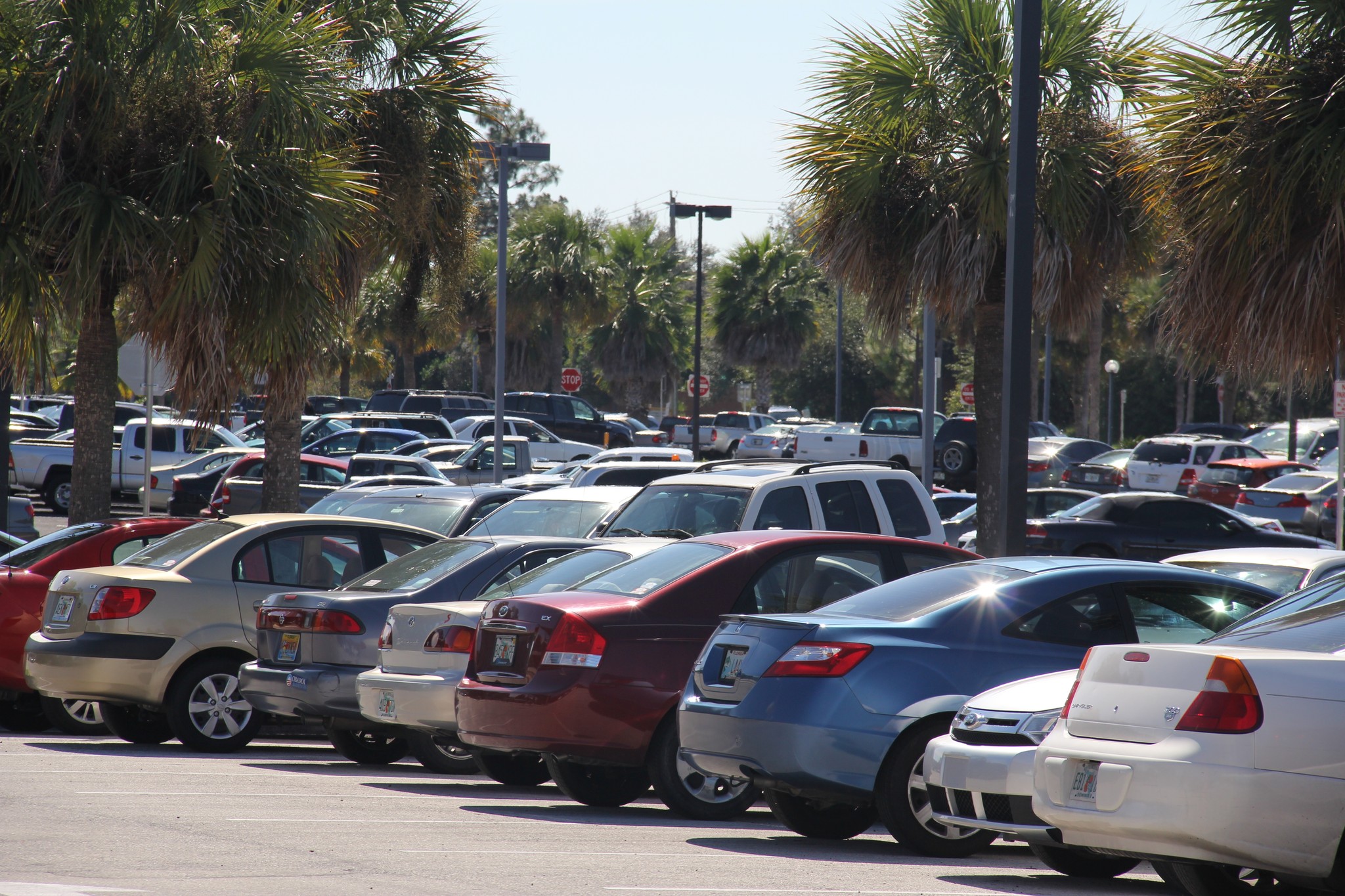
[685,372,713,397]
[559,367,582,392]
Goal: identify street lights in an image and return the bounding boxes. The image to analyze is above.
[469,138,551,489]
[1105,357,1122,446]
[668,202,734,463]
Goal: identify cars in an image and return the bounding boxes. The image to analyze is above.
[1,385,1344,894]
